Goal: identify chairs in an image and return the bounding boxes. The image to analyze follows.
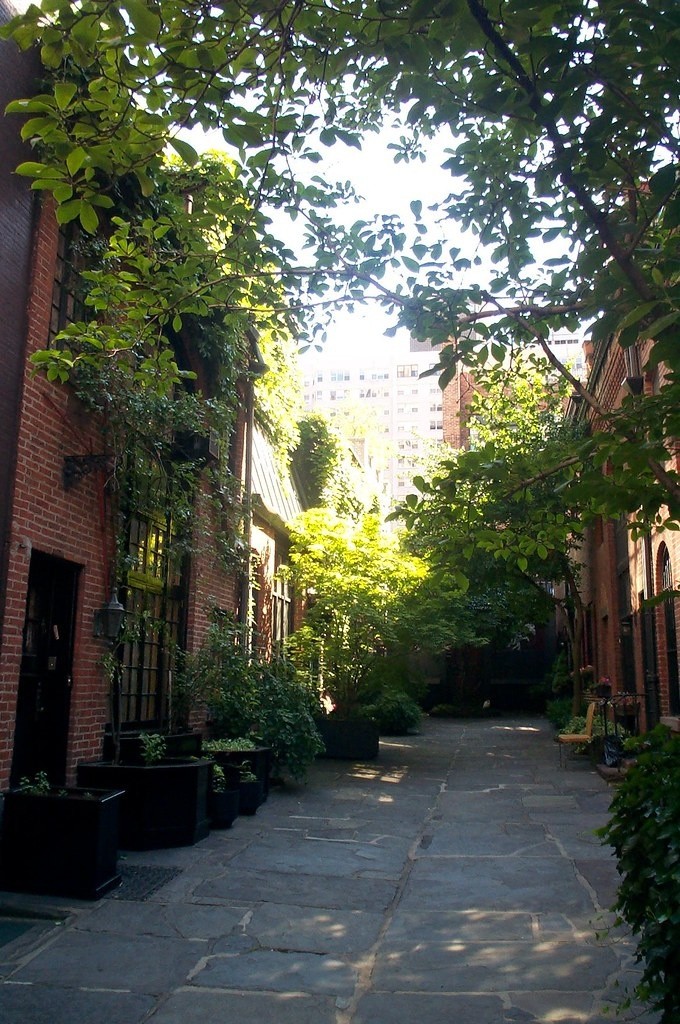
[558,701,595,768]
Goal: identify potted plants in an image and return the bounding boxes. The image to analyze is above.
[77,730,217,852]
[312,592,382,760]
[200,756,239,830]
[0,771,127,901]
[200,738,271,804]
[238,772,261,817]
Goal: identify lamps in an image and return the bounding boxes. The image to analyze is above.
[99,587,125,639]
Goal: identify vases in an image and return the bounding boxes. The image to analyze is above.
[596,686,612,696]
[616,702,640,716]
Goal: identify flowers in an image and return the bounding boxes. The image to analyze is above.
[617,691,638,706]
[594,677,610,687]
